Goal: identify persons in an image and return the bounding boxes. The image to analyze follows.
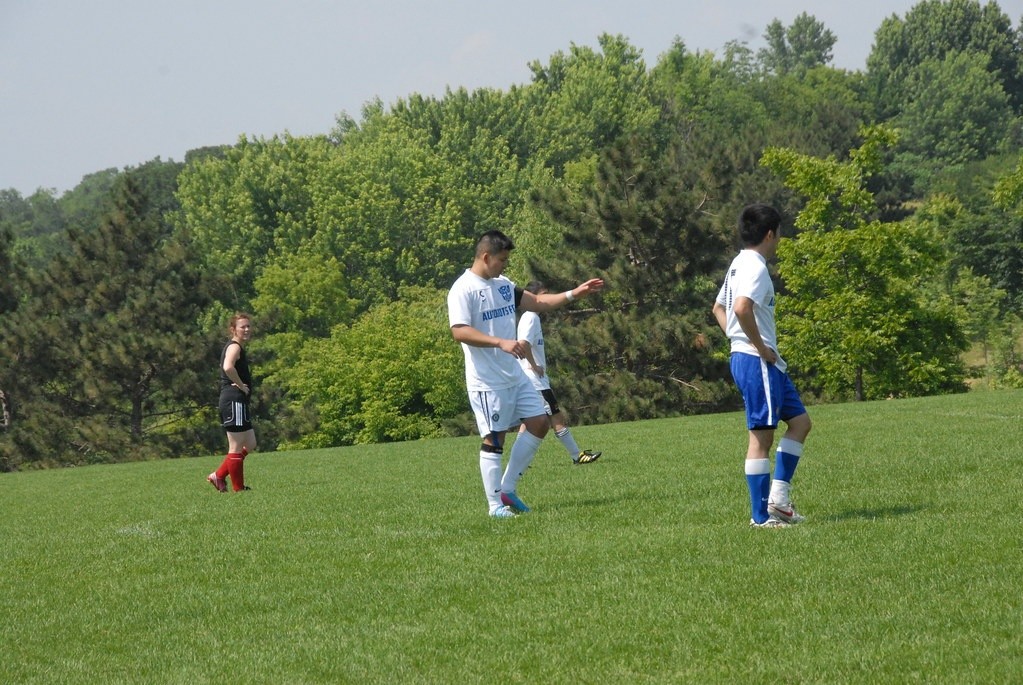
[447,230,604,520]
[712,205,812,530]
[207,314,256,493]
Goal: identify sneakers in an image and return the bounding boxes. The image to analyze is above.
[233,485,251,493]
[767,504,805,524]
[749,517,791,529]
[501,491,528,512]
[573,449,601,464]
[208,471,228,492]
[489,505,516,517]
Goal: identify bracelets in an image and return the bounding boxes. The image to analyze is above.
[565,290,575,302]
